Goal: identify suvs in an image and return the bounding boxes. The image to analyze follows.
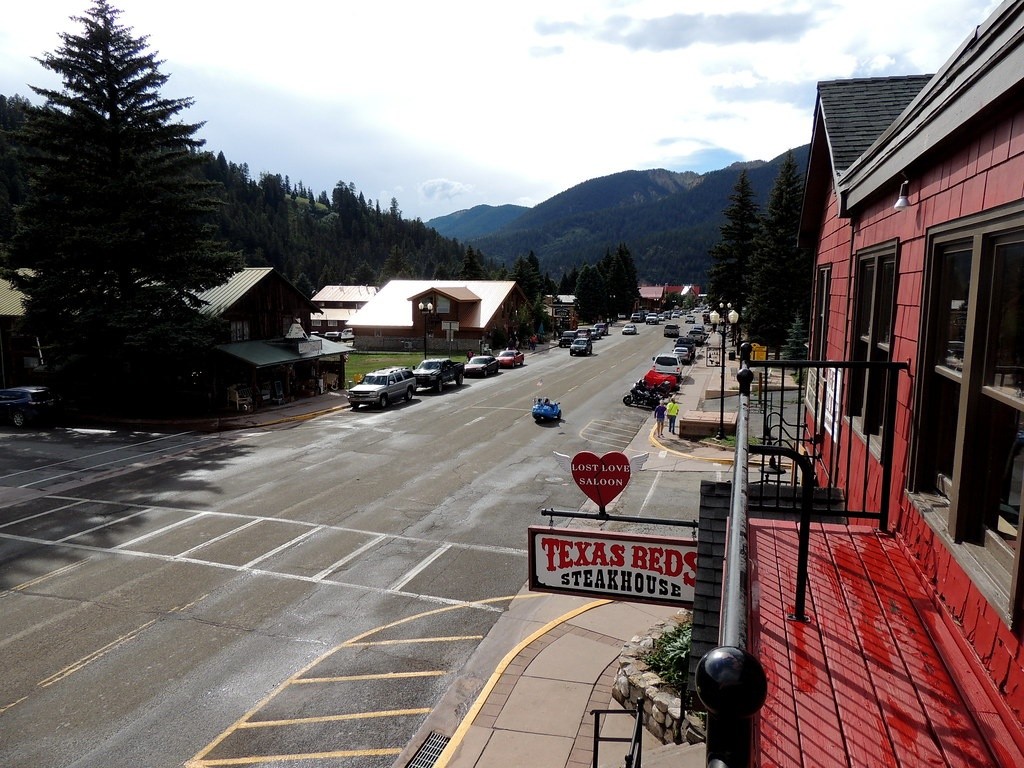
[346,366,418,411]
[569,337,592,357]
[0,386,60,431]
[651,353,683,384]
[558,331,578,348]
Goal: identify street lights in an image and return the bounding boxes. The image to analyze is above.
[709,301,740,440]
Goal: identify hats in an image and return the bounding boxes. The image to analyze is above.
[659,400,664,404]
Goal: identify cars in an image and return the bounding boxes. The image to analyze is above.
[462,355,501,378]
[672,347,692,365]
[575,323,608,339]
[622,324,637,335]
[310,327,356,342]
[630,307,711,359]
[495,349,525,369]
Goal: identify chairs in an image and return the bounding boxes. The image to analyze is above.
[227,390,247,411]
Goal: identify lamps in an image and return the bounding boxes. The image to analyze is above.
[894,171,911,211]
[756,412,821,474]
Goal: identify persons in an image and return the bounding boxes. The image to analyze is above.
[545,398,554,409]
[554,329,556,340]
[466,349,475,360]
[654,400,667,437]
[666,398,679,434]
[480,341,485,356]
[508,328,537,350]
[537,398,544,406]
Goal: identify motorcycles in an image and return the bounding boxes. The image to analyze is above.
[622,378,670,411]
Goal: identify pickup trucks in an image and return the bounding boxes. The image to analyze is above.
[411,358,463,394]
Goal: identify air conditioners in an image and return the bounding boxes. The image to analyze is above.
[404,342,412,348]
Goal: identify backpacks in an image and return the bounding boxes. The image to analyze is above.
[469,351,472,357]
